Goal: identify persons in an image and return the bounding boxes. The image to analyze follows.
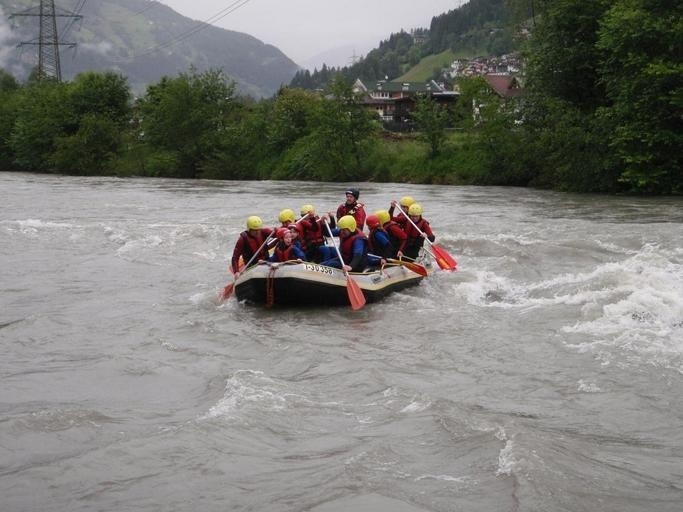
[396,195,415,215]
[278,204,407,272]
[257,227,305,265]
[230,215,276,281]
[387,200,435,264]
[335,187,366,231]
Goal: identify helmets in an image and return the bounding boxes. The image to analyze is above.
[301,205,315,216]
[337,215,357,232]
[278,208,296,224]
[276,228,289,239]
[247,216,263,229]
[408,204,422,215]
[400,197,415,207]
[366,216,380,229]
[377,210,391,225]
[345,188,359,199]
[288,223,301,232]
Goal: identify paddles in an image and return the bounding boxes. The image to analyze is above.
[394,203,457,272]
[366,251,428,278]
[223,231,274,299]
[230,212,308,273]
[324,218,366,310]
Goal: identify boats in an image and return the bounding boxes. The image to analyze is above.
[233,258,426,306]
